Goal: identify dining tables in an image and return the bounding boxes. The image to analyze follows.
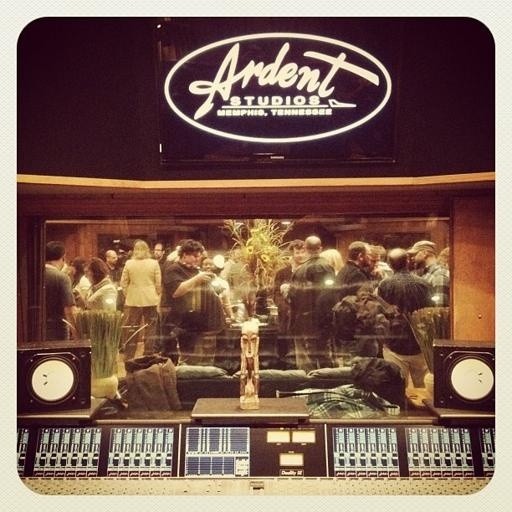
[216,217,293,315]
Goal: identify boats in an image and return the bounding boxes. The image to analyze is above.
[17,340,92,414]
[432,339,495,411]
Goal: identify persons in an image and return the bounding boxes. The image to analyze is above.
[61,250,133,314]
[163,240,234,366]
[271,236,342,374]
[119,239,162,361]
[45,242,75,343]
[332,240,394,359]
[404,247,425,277]
[153,243,166,269]
[407,240,449,309]
[379,248,434,409]
